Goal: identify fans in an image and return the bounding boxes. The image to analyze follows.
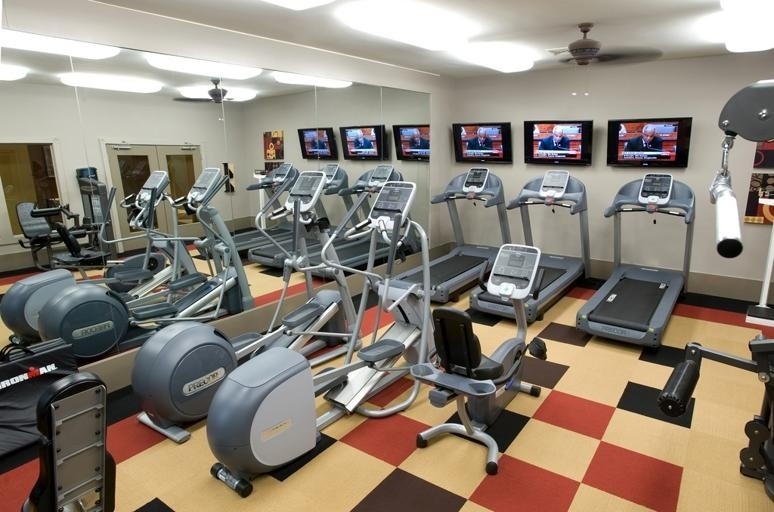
[173,78,256,104]
[525,24,665,66]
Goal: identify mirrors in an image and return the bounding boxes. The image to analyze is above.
[0,28,431,370]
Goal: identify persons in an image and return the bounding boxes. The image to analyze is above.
[409,128,429,148]
[311,132,326,149]
[625,125,663,151]
[353,129,374,149]
[467,127,493,150]
[274,139,283,159]
[539,127,570,150]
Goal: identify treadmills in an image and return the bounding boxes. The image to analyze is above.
[468,169,593,324]
[402,167,512,306]
[575,172,696,348]
[210,162,419,279]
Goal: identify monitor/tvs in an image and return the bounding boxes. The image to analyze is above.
[339,124,388,161]
[451,122,512,164]
[297,127,338,160]
[523,120,594,166]
[607,117,693,168]
[392,124,430,162]
